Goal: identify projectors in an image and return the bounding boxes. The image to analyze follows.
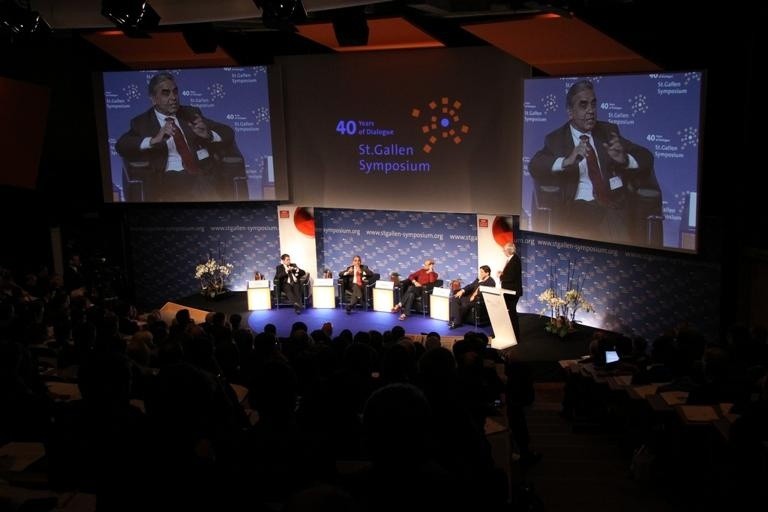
[100,0,162,40]
[0,1,56,41]
[251,0,309,36]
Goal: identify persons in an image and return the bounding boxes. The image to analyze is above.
[529,80,656,242]
[495,243,523,339]
[114,71,238,201]
[3,251,766,512]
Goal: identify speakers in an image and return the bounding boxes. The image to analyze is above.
[331,10,370,47]
[182,24,218,54]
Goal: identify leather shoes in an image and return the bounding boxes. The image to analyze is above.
[294,302,303,315]
[346,304,353,315]
[448,322,463,329]
[392,305,406,321]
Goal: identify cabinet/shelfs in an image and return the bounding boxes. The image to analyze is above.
[312,278,339,309]
[246,287,272,311]
[372,285,400,313]
[429,293,461,322]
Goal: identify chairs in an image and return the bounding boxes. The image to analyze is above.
[531,172,663,249]
[336,274,380,312]
[449,292,492,330]
[272,272,311,310]
[122,136,251,202]
[399,278,443,318]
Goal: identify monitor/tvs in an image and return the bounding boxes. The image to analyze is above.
[603,350,620,364]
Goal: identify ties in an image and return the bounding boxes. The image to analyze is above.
[165,117,194,171]
[357,271,362,287]
[287,266,294,287]
[580,134,611,205]
[469,288,478,302]
